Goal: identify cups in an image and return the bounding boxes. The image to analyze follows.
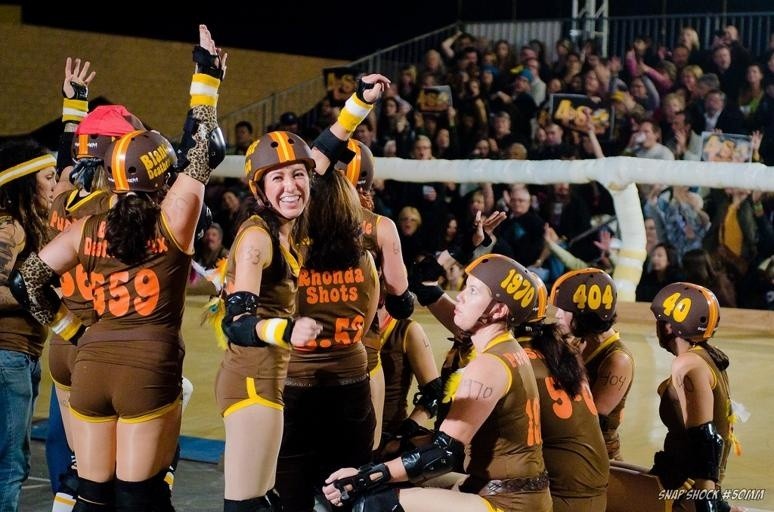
[635,133,647,145]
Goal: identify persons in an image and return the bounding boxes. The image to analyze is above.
[1,24,772,511]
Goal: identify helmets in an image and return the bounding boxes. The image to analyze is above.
[244,130,317,212]
[69,103,146,166]
[101,128,179,193]
[651,281,721,344]
[525,272,547,324]
[464,253,537,330]
[550,267,618,337]
[338,138,376,195]
